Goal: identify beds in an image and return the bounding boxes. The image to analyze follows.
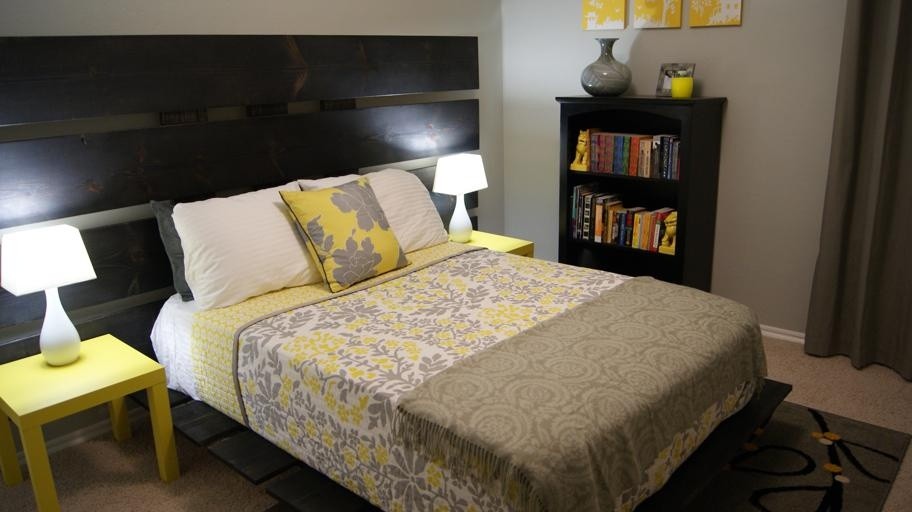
[0,36,794,511]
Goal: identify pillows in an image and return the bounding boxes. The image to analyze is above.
[149,187,273,301]
[298,168,450,254]
[278,176,413,293]
[171,180,323,311]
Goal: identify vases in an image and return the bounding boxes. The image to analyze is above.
[581,37,631,97]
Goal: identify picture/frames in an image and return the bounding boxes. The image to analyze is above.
[655,62,696,97]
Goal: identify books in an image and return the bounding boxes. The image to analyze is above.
[568,128,680,252]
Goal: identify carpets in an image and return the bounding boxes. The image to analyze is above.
[265,401,912,512]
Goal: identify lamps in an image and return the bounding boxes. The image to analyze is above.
[432,153,488,242]
[0,223,97,366]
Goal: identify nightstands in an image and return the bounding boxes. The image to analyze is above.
[447,230,534,259]
[1,332,180,511]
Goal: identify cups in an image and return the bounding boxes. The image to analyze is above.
[671,73,694,98]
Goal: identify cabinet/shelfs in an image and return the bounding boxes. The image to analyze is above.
[555,96,728,293]
